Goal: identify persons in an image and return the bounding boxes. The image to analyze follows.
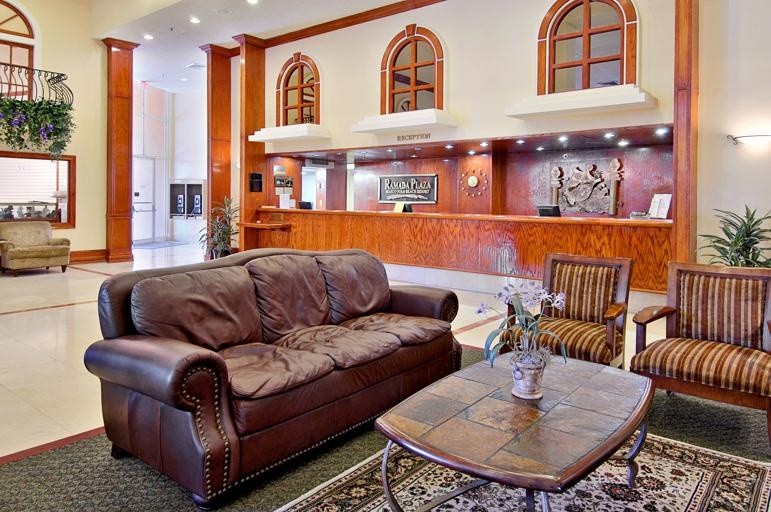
[43,205,50,216]
[4,205,13,218]
[18,207,23,217]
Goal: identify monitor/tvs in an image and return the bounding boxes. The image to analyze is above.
[402,204,413,212]
[298,201,311,209]
[536,206,562,217]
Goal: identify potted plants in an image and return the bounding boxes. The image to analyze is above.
[196,194,240,259]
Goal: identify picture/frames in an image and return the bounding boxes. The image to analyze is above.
[376,173,439,205]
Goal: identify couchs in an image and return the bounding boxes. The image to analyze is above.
[80,246,463,511]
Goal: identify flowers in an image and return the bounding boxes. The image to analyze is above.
[0,95,76,163]
[477,266,570,372]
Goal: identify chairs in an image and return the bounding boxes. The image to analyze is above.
[499,250,633,371]
[630,259,771,441]
[0,221,73,277]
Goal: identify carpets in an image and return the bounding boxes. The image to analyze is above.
[0,342,770,512]
